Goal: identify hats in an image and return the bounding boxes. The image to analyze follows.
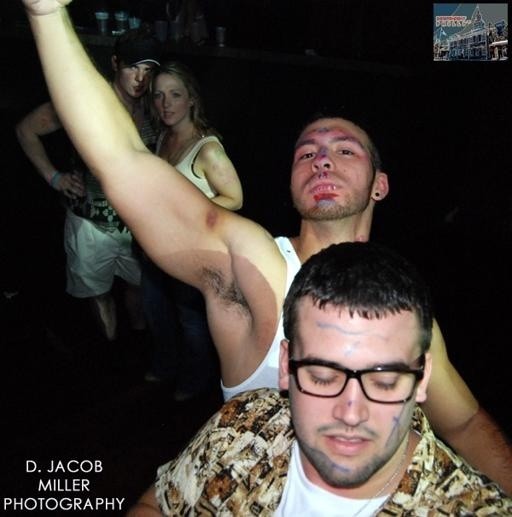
[110,27,161,68]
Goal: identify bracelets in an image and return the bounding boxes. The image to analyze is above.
[50,169,64,189]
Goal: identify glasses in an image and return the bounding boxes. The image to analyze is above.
[286,339,426,404]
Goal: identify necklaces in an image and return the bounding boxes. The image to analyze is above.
[163,131,204,165]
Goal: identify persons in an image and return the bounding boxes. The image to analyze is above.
[121,241,512,517]
[21,0,512,496]
[13,22,165,361]
[133,59,245,401]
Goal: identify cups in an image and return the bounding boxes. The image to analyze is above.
[93,9,227,49]
